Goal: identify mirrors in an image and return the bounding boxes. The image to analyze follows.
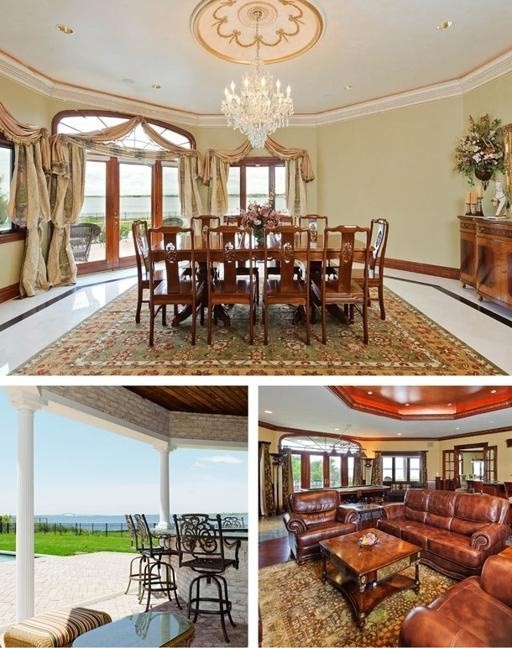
[454,442,488,488]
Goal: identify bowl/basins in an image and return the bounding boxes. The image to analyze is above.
[358,536,381,547]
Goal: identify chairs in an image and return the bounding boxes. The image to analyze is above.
[123,513,246,642]
[70,224,94,262]
[133,212,390,346]
[282,489,358,565]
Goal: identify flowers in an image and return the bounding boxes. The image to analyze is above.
[452,112,506,190]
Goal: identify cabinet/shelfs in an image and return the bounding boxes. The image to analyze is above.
[458,214,511,312]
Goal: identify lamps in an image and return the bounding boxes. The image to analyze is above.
[223,15,294,152]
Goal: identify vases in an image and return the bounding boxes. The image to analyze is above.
[474,165,493,183]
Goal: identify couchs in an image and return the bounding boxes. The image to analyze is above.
[376,486,510,583]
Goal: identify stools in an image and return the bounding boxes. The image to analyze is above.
[402,547,512,648]
[4,606,112,648]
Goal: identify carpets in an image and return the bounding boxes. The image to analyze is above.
[260,560,456,647]
[10,282,508,376]
